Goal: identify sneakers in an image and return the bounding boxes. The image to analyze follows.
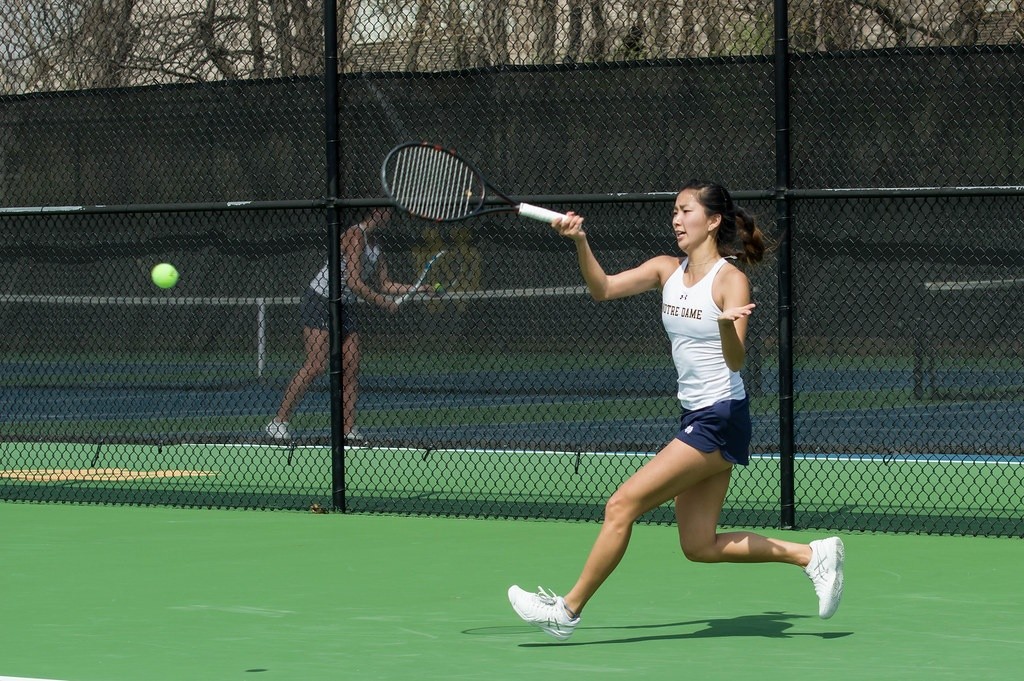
[266,418,292,440]
[804,535,846,618]
[343,428,364,440]
[506,585,582,641]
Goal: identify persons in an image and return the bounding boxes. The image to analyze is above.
[505,178,845,640]
[264,200,439,446]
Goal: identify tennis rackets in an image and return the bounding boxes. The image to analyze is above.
[394,248,465,308]
[378,139,584,231]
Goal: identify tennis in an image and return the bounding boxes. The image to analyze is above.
[434,283,444,294]
[151,263,180,290]
